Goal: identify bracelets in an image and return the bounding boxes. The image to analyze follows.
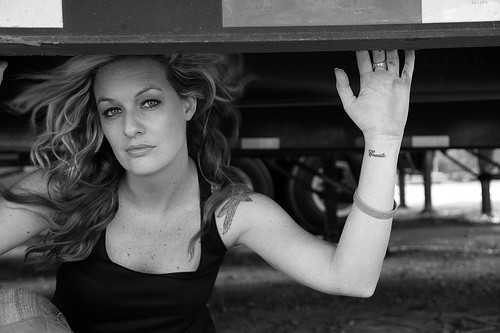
[352,187,398,220]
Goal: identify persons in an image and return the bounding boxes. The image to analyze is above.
[0,50,416,333]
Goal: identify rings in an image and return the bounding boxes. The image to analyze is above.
[373,61,386,69]
